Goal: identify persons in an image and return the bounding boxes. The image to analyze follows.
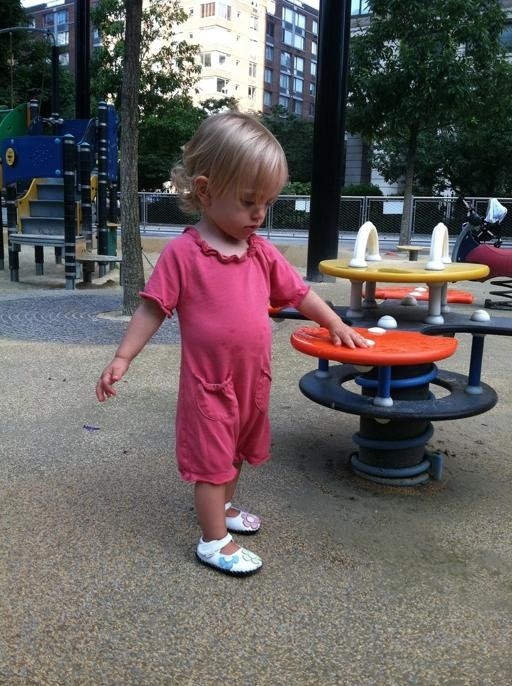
[96,112,371,576]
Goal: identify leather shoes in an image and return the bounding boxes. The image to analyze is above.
[197,532,263,581]
[222,502,263,534]
